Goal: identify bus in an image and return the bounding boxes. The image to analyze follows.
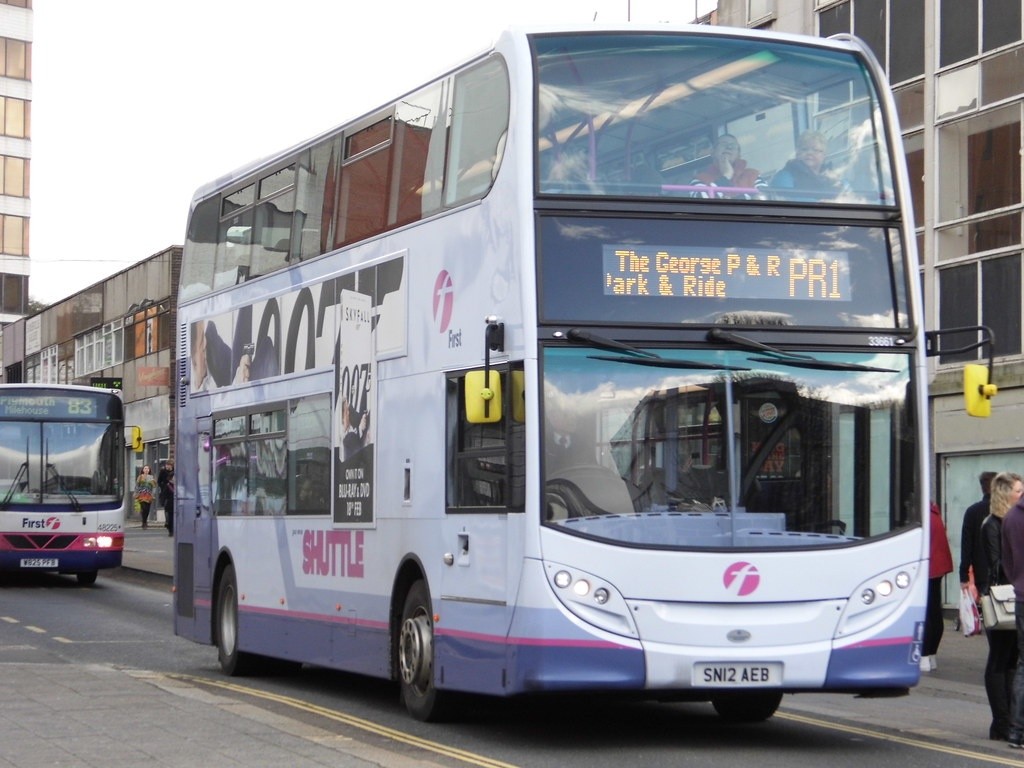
[0,381,144,589]
[171,23,1001,727]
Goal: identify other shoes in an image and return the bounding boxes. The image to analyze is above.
[920,655,937,672]
[142,523,147,530]
[1008,733,1024,748]
[990,724,1009,741]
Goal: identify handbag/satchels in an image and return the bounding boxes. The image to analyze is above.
[159,493,166,506]
[134,500,143,513]
[164,498,172,512]
[959,587,982,637]
[981,584,1017,630]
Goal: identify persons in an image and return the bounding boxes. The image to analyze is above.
[688,135,768,200]
[544,390,599,482]
[190,316,250,394]
[334,392,368,465]
[296,477,320,512]
[768,129,854,203]
[130,458,176,538]
[916,471,1024,748]
